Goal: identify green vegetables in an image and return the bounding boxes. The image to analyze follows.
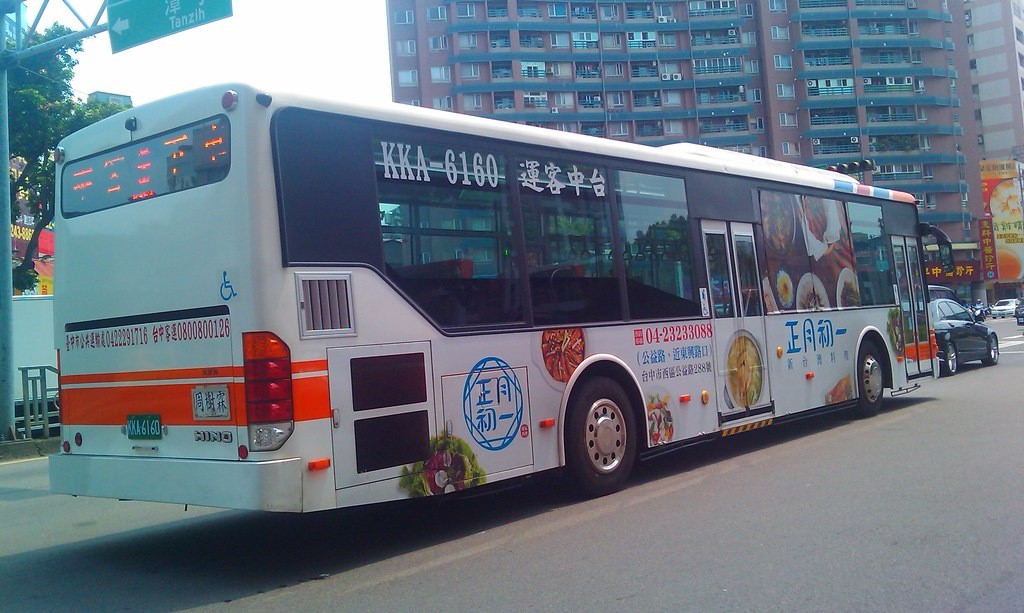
[398,433,487,499]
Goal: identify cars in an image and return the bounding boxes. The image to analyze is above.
[1014,300,1024,324]
[930,299,999,374]
[992,299,1021,319]
[926,285,968,308]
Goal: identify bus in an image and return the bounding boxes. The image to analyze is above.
[48,80,955,515]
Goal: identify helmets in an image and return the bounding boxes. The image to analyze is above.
[977,299,981,302]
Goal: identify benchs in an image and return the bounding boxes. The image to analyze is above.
[396,260,472,330]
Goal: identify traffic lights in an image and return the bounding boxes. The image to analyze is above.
[828,159,876,174]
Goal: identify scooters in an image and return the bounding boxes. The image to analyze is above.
[970,304,986,322]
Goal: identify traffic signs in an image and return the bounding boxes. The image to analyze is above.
[106,0,234,54]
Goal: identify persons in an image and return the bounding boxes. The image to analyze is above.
[976,299,987,321]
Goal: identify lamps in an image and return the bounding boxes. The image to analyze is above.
[497,104,504,110]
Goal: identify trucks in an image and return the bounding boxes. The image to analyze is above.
[13,295,61,434]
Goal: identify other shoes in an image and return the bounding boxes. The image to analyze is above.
[985,318,987,321]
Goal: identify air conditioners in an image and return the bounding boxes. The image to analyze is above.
[873,165,881,173]
[978,137,982,143]
[965,222,970,230]
[807,80,817,88]
[658,17,667,23]
[662,74,670,81]
[673,74,681,80]
[546,67,554,75]
[908,2,916,9]
[813,139,820,146]
[904,76,913,84]
[593,97,601,101]
[962,193,968,201]
[886,77,895,84]
[851,137,858,143]
[552,107,558,113]
[728,30,735,35]
[869,137,876,146]
[863,78,871,84]
